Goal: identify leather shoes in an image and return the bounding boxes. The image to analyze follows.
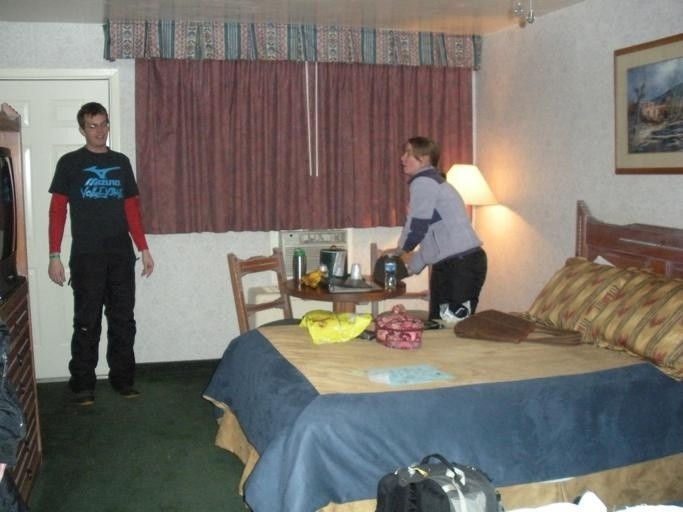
[75,392,95,406]
[112,384,141,398]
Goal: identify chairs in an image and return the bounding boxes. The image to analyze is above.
[369,243,431,321]
[226,248,292,334]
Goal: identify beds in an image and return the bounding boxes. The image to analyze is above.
[231,201,682,512]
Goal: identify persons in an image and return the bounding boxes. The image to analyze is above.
[45,101,155,408]
[381,136,486,329]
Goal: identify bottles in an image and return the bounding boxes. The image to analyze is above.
[383,253,397,291]
[292,247,308,283]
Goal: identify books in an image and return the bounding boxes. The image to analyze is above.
[326,283,376,294]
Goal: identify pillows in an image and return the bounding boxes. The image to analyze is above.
[519,254,681,383]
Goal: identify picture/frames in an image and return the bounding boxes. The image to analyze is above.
[612,32,682,174]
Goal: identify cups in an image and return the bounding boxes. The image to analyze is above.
[350,262,362,281]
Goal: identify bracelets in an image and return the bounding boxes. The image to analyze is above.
[48,254,60,260]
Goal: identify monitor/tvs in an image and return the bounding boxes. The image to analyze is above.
[0,146,19,304]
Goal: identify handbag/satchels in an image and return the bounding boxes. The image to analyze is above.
[375,454,505,512]
[453,309,582,347]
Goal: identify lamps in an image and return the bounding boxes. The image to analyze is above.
[445,163,494,207]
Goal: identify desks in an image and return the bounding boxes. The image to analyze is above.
[284,275,405,313]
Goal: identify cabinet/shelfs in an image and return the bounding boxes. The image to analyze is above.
[0,279,42,506]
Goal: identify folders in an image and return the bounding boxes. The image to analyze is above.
[327,278,371,294]
